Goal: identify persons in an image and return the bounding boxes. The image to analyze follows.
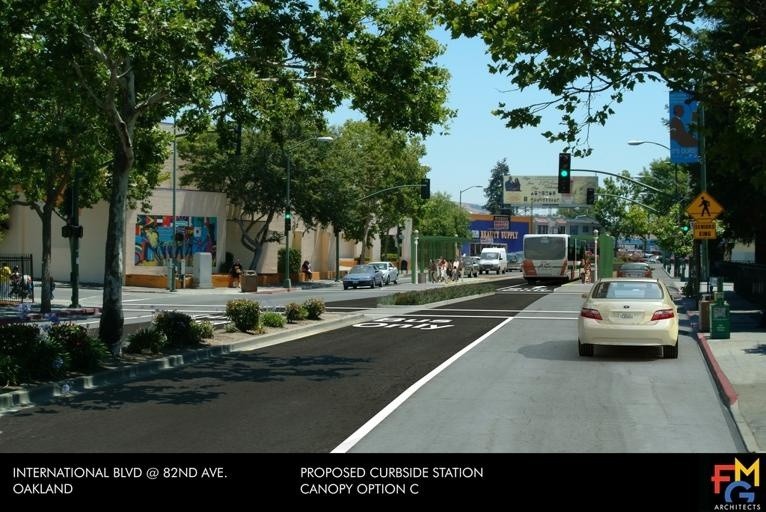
[302,261,312,281]
[430,256,463,285]
[232,260,242,288]
[307,263,311,271]
[579,258,592,284]
[0,263,56,300]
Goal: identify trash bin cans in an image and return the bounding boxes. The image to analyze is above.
[699,294,715,331]
[241,270,258,291]
